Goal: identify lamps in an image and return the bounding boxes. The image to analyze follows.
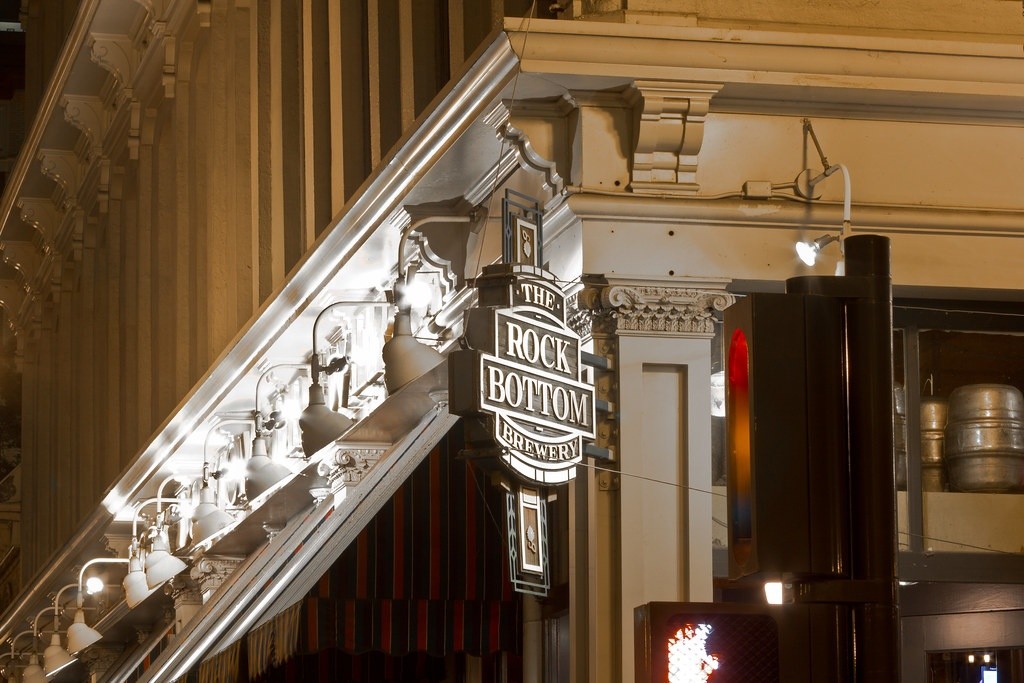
[834,240,846,277]
[20,605,98,683]
[66,557,131,656]
[382,206,489,397]
[143,471,225,590]
[247,356,348,503]
[43,582,123,677]
[123,497,187,609]
[794,234,839,265]
[192,418,283,544]
[299,301,390,458]
